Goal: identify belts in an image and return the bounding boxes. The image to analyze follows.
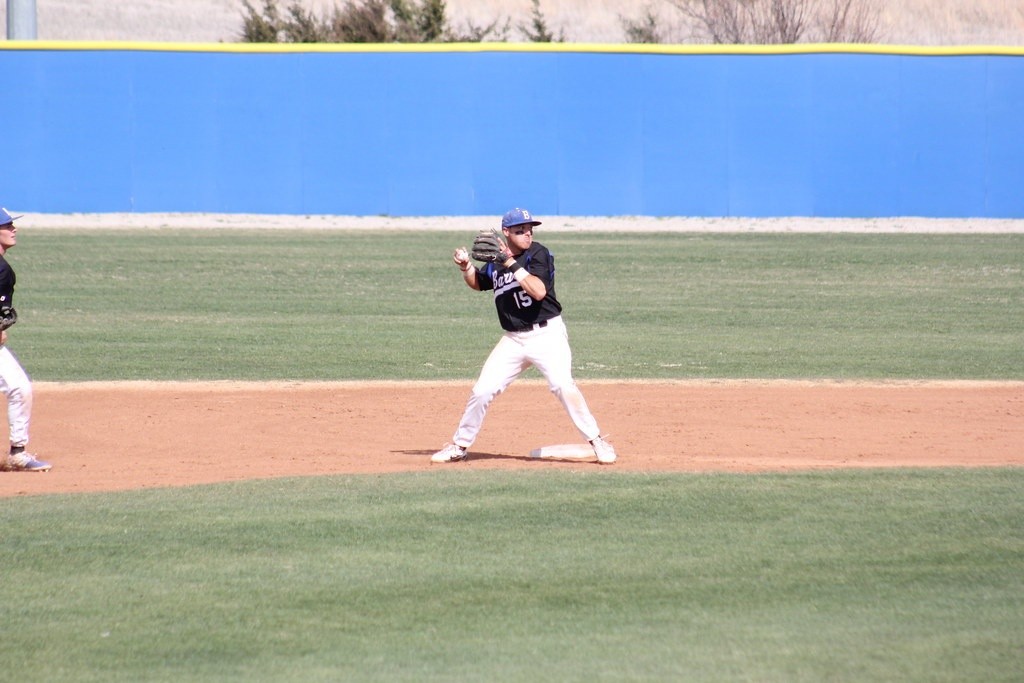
[510,320,547,332]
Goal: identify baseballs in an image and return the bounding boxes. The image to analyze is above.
[457,250,469,262]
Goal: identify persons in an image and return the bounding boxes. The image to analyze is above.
[431,207,616,464]
[0,207,52,470]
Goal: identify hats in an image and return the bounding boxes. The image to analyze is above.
[0,207,24,225]
[502,208,542,227]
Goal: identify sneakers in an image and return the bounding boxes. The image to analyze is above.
[3,451,52,472]
[431,444,468,462]
[592,434,616,463]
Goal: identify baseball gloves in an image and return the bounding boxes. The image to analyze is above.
[0,304,17,332]
[471,226,512,265]
